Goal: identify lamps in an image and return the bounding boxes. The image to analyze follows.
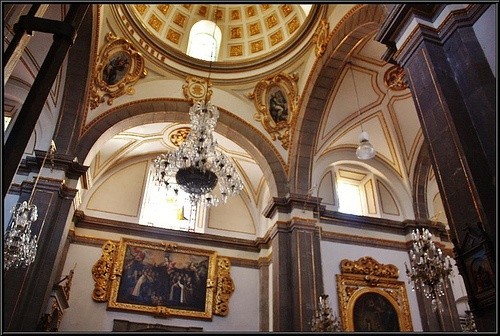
[4,145,53,272]
[348,60,375,160]
[153,3,244,207]
[405,227,454,315]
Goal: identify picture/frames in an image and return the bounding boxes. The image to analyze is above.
[106,237,217,319]
